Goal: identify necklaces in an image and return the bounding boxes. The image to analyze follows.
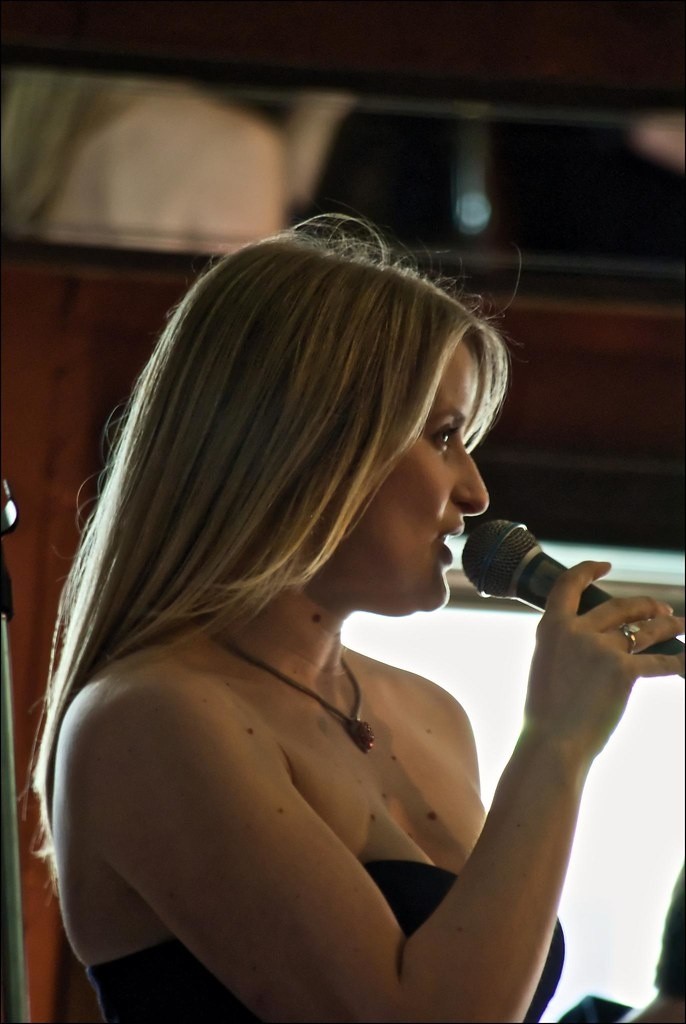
[218,630,375,751]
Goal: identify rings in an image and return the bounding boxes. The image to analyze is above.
[619,623,637,653]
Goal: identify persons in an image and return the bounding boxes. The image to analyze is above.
[27,225,686,1024]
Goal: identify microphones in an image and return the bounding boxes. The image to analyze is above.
[462,519,686,681]
